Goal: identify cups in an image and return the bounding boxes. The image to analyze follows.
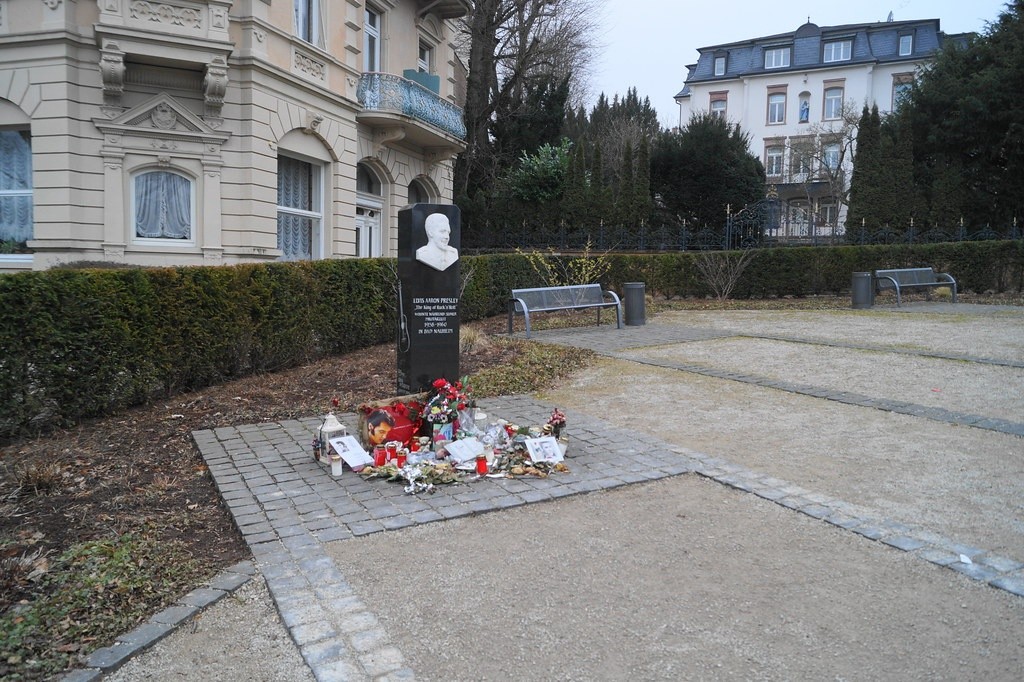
[477,454,487,474]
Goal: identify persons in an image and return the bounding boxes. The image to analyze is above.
[366,409,396,445]
[337,441,348,452]
[800,100,809,120]
[541,442,555,459]
[416,213,459,272]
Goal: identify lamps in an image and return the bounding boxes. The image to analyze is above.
[317,412,346,464]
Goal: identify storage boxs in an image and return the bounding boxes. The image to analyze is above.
[367,402,424,446]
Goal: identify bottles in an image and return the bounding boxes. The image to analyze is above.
[387,445,397,462]
[397,451,406,468]
[411,436,420,451]
[331,455,343,476]
[375,445,385,466]
[318,412,346,465]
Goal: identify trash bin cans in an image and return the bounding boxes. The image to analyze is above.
[851,271,872,309]
[624,282,645,325]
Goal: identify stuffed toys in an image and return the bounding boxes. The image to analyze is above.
[415,436,431,452]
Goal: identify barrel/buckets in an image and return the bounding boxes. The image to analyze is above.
[624,282,646,326]
[851,272,871,309]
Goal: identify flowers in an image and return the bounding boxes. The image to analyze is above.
[392,375,478,424]
[547,407,566,436]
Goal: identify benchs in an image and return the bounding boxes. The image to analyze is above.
[508,283,623,339]
[872,267,957,307]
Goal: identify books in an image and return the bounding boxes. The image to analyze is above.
[445,436,486,462]
